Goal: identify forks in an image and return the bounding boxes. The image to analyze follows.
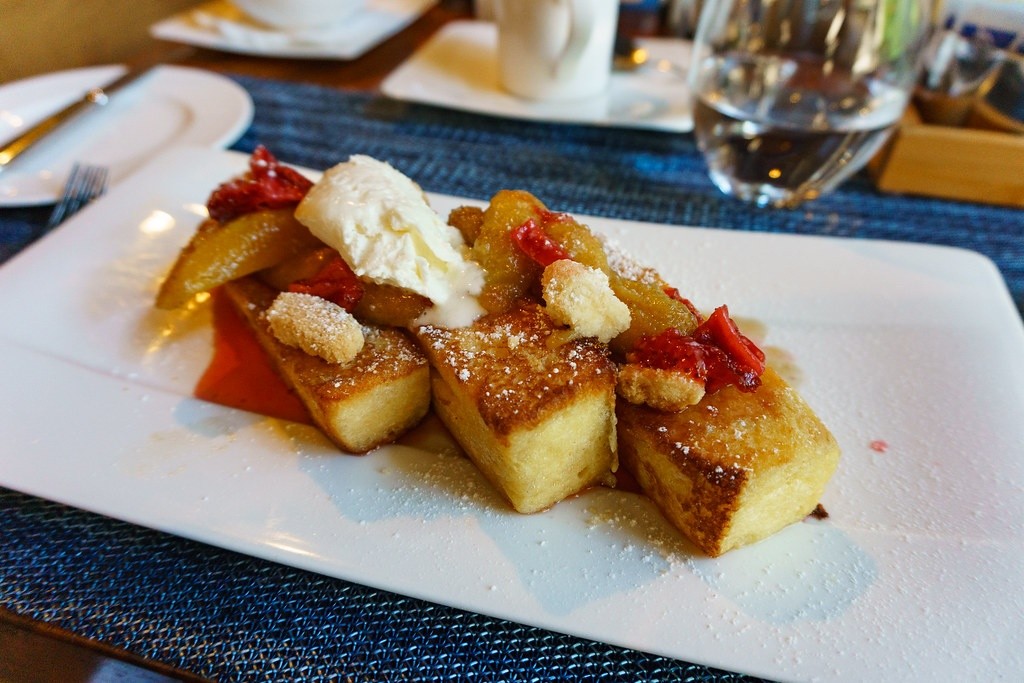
[39,161,111,238]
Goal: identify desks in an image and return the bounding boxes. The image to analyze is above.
[0,1,1024,683]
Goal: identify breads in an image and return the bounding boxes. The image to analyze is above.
[223,272,840,553]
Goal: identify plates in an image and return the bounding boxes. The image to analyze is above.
[0,62,256,207]
[378,16,713,135]
[0,140,1024,683]
[145,0,440,62]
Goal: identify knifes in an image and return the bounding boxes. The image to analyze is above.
[0,40,210,170]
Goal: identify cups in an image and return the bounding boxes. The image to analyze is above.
[494,1,620,102]
[685,0,948,212]
[227,1,370,35]
